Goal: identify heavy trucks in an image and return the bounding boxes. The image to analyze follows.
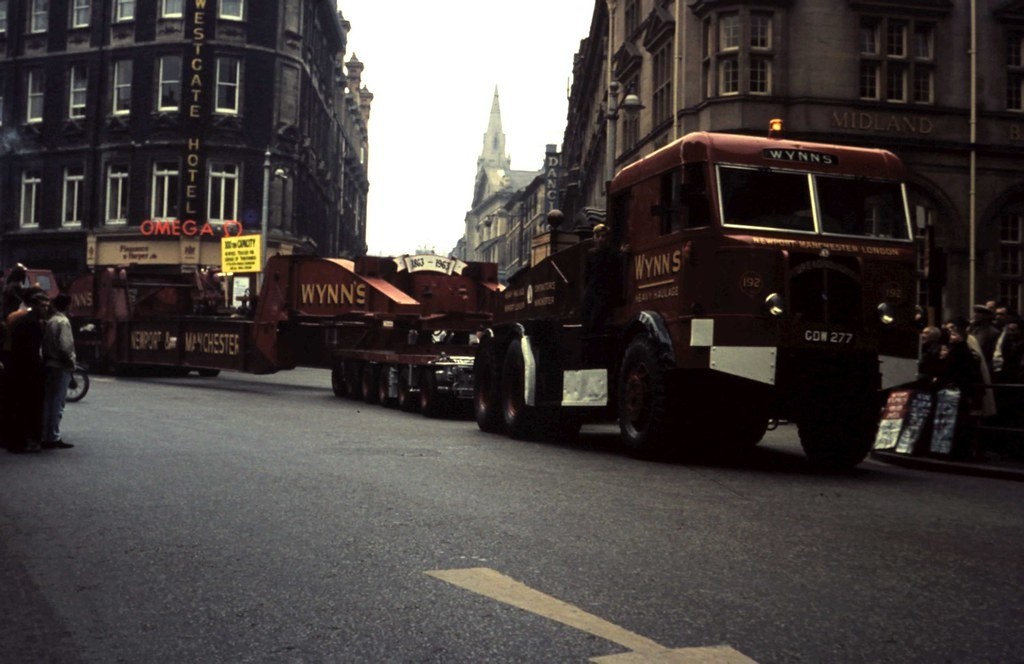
[57,117,937,466]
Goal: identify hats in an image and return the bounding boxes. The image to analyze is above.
[973,304,993,315]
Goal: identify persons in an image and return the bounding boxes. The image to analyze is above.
[0,263,78,455]
[917,300,1024,417]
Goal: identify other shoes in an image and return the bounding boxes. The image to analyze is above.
[43,437,75,449]
[10,440,41,453]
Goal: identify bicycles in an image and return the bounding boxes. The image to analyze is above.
[61,364,89,402]
